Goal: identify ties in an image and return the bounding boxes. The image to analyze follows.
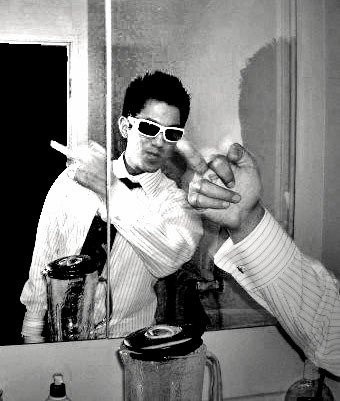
[79,176,142,278]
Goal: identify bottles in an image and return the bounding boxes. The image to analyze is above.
[45,374,71,401]
[283,359,334,401]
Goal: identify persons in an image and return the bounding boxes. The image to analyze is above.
[174,138,340,379]
[18,68,206,347]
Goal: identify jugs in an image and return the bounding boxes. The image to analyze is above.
[117,323,223,401]
[42,254,113,343]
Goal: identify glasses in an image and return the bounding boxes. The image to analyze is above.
[124,116,188,145]
